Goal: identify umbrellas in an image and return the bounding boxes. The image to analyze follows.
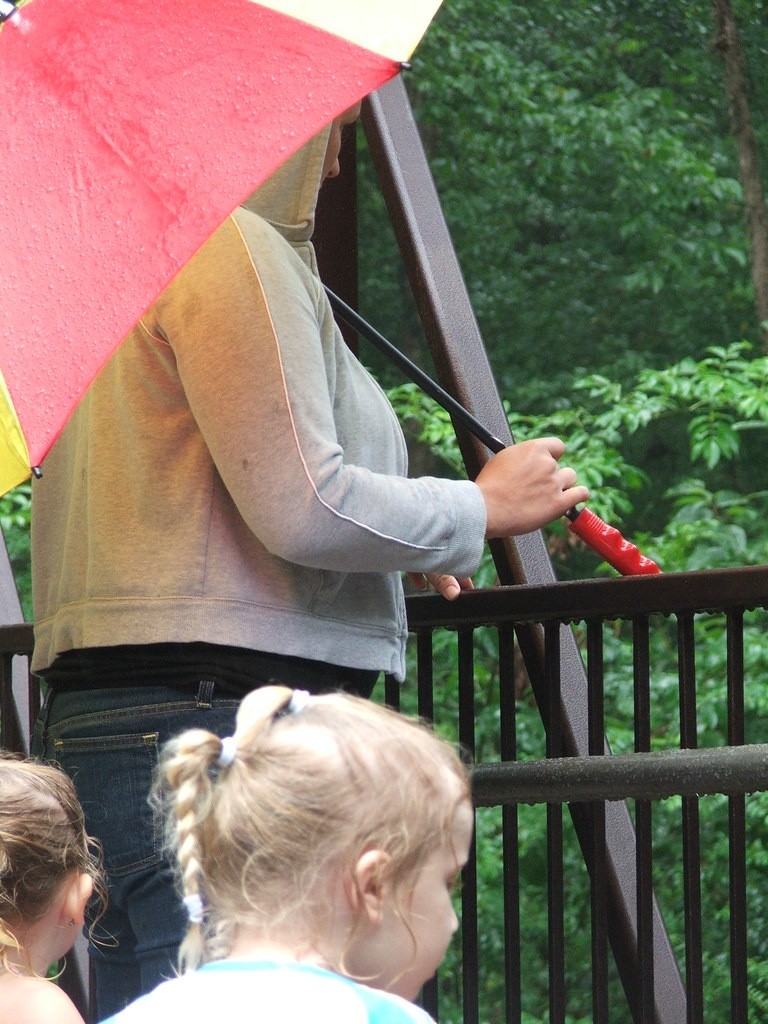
[0,0,659,577]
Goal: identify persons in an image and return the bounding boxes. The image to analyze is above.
[0,760,95,1024]
[100,686,474,1024]
[35,94,588,1024]
